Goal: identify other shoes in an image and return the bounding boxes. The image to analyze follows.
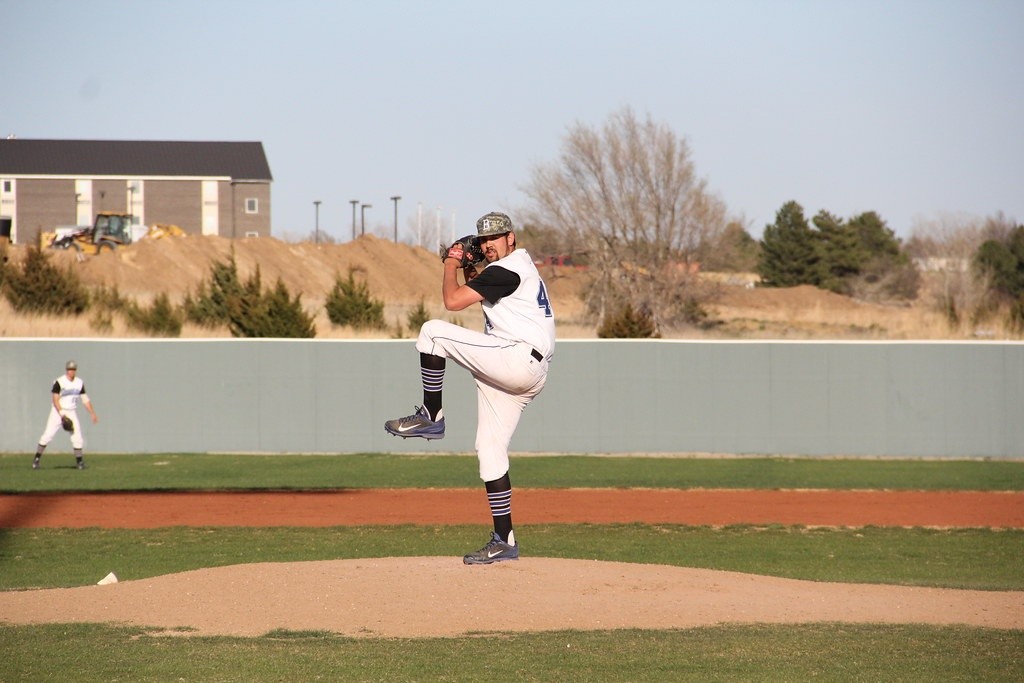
[32,457,39,469]
[77,461,84,470]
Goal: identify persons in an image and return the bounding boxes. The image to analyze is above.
[384,211,554,563]
[32,360,96,469]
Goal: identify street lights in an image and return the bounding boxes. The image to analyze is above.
[390,196,401,243]
[313,200,322,244]
[360,204,369,237]
[349,201,360,239]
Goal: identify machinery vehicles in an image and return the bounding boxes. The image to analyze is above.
[39,210,188,255]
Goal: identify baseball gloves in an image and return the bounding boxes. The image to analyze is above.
[61,414,74,434]
[439,234,486,269]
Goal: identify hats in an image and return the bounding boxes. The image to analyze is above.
[66,362,76,370]
[476,212,513,237]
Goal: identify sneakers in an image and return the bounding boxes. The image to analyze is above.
[384,404,445,441]
[463,531,518,565]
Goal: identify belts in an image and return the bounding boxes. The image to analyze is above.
[530,348,543,362]
